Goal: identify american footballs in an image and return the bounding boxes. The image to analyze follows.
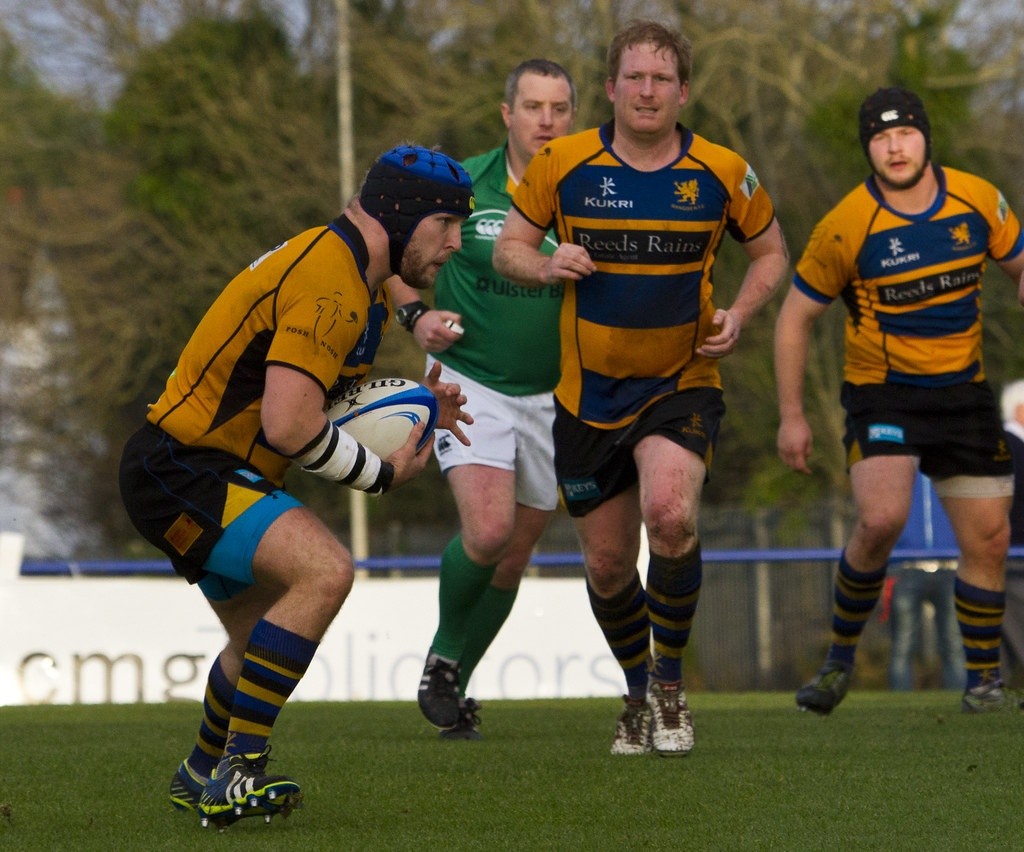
[322,377,440,461]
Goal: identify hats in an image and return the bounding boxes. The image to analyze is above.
[359,145,475,241]
[859,86,932,154]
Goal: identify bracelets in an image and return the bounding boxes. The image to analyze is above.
[406,306,433,334]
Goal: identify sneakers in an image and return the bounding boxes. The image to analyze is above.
[796,659,851,713]
[609,694,652,756]
[961,673,1004,714]
[647,681,695,756]
[197,750,303,834]
[418,647,461,730]
[171,756,208,809]
[439,697,482,741]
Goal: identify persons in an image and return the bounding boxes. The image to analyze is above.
[493,20,791,758]
[773,88,1024,718]
[118,143,474,835]
[381,60,580,729]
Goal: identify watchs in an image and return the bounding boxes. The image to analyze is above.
[395,300,424,325]
